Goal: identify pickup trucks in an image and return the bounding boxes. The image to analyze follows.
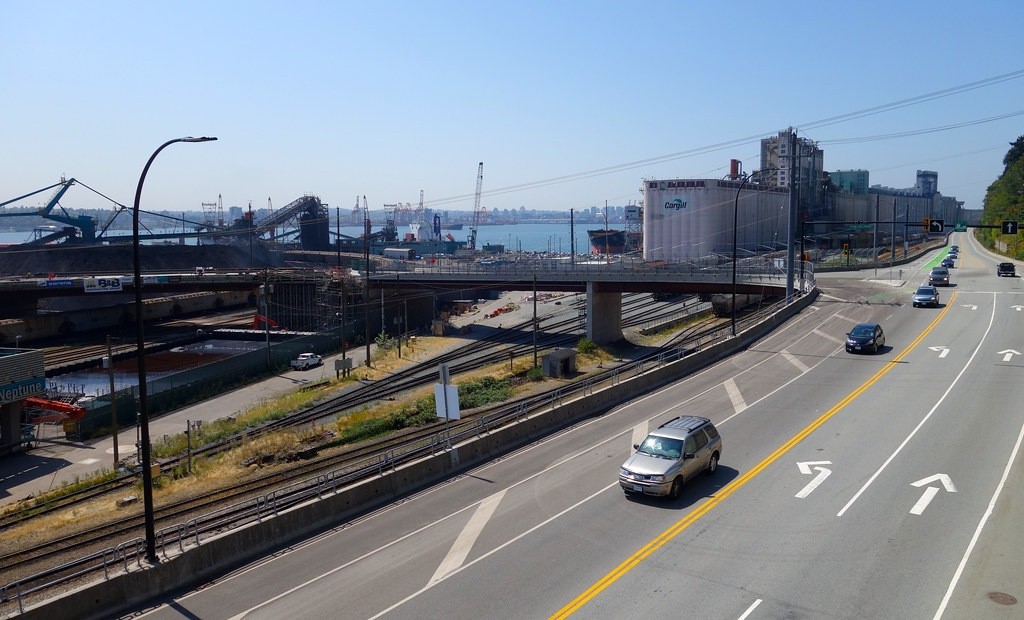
[291,352,322,370]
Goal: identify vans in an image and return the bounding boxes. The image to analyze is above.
[929,267,949,286]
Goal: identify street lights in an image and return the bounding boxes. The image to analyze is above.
[133,136,217,564]
[732,167,791,334]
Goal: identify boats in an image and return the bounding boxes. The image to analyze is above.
[586,229,641,254]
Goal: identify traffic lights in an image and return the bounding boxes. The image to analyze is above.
[844,244,848,255]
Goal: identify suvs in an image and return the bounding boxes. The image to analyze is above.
[619,415,722,500]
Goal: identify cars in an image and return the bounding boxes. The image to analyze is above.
[941,258,954,268]
[845,323,886,354]
[997,263,1015,276]
[947,251,957,259]
[912,286,939,307]
[951,246,959,252]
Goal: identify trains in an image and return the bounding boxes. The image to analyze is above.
[0,290,258,345]
[699,251,789,302]
[651,245,783,301]
[711,249,823,316]
